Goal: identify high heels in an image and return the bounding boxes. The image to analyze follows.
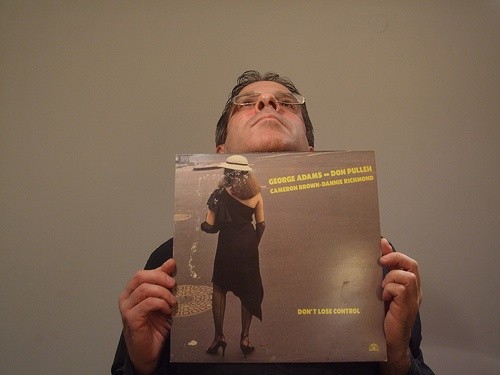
[206,342,227,357]
[240,345,255,357]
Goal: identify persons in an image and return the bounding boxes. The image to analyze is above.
[200,155,265,357]
[112,68,435,375]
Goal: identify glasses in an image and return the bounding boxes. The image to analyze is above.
[227,91,307,106]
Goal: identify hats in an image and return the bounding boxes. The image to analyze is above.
[218,155,252,172]
